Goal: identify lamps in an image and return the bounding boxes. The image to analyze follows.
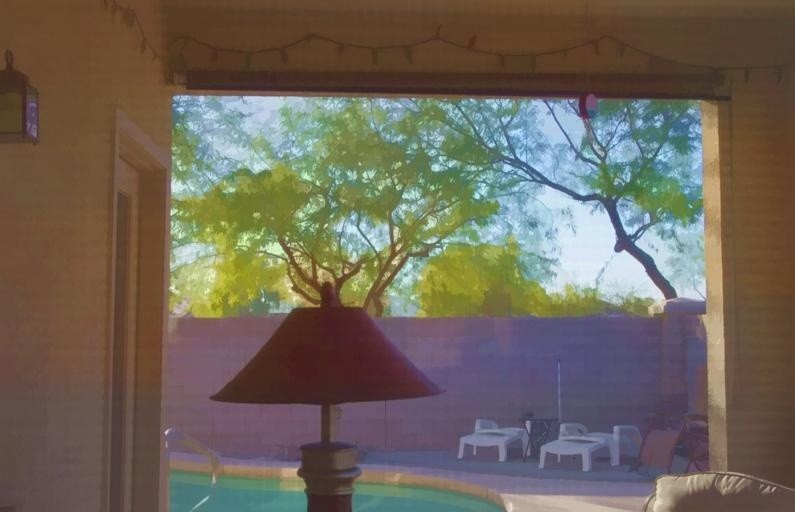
[211,285,441,510]
[1,49,42,146]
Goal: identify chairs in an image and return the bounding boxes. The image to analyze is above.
[455,415,708,479]
